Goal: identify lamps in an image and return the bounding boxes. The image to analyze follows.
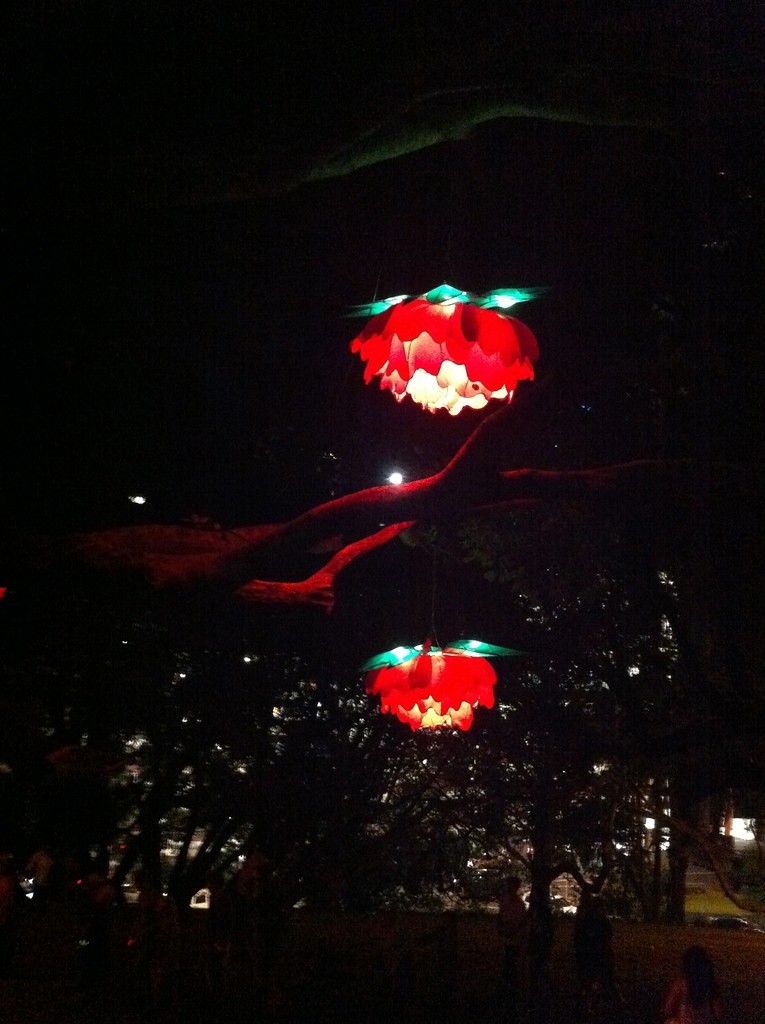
[352,534,515,734]
[342,221,539,415]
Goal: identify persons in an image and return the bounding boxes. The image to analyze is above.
[563,892,613,988]
[499,878,526,927]
[663,941,723,1023]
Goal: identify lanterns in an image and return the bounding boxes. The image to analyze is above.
[339,285,542,418]
[356,637,527,733]
